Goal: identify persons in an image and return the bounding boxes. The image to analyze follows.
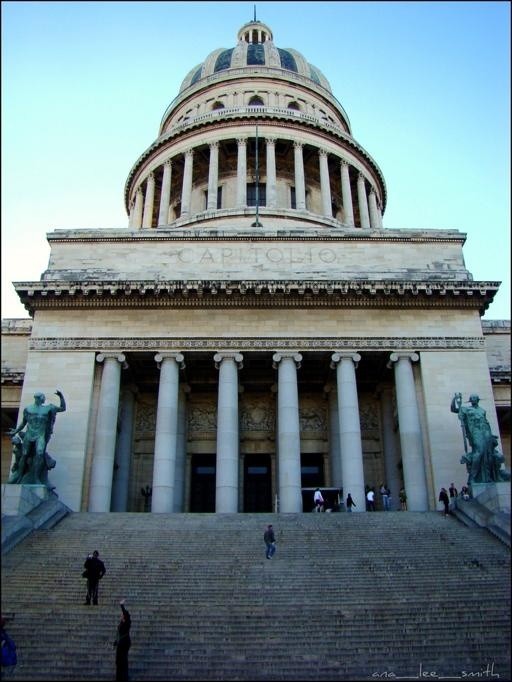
[313,487,324,511]
[112,599,133,682]
[449,482,458,508]
[346,492,356,511]
[437,487,449,517]
[379,484,392,511]
[459,487,471,501]
[450,392,493,484]
[367,484,376,510]
[398,489,408,511]
[9,427,56,476]
[83,549,106,603]
[333,489,340,512]
[263,524,276,559]
[4,389,67,483]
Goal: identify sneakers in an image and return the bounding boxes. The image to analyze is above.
[83,601,99,606]
[267,555,273,559]
